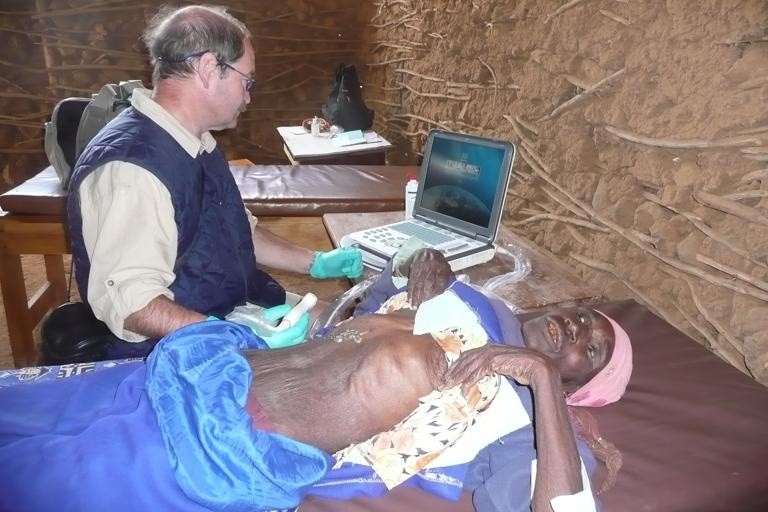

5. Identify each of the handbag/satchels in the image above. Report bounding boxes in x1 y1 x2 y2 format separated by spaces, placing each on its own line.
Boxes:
321 63 374 131
43 78 145 191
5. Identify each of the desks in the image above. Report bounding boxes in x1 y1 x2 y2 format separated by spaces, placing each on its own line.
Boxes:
321 204 603 316
273 119 396 167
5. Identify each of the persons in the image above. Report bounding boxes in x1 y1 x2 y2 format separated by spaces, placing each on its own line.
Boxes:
64 0 364 361
0 246 635 512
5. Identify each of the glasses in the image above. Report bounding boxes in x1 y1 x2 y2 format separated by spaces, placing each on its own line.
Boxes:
176 50 257 92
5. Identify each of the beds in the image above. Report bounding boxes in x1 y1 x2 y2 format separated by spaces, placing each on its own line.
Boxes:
0 152 416 364
0 301 766 511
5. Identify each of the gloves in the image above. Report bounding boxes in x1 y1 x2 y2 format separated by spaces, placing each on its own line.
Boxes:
202 303 311 348
308 244 366 283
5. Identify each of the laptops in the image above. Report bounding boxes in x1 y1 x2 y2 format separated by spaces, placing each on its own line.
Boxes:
340 128 517 273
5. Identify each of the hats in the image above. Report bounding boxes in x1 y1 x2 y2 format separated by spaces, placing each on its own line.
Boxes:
564 308 636 409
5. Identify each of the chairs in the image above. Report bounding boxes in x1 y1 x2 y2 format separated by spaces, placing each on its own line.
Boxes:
36 97 143 363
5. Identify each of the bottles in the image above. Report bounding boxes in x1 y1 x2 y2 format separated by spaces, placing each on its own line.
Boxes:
311 116 320 137
404 177 420 219
